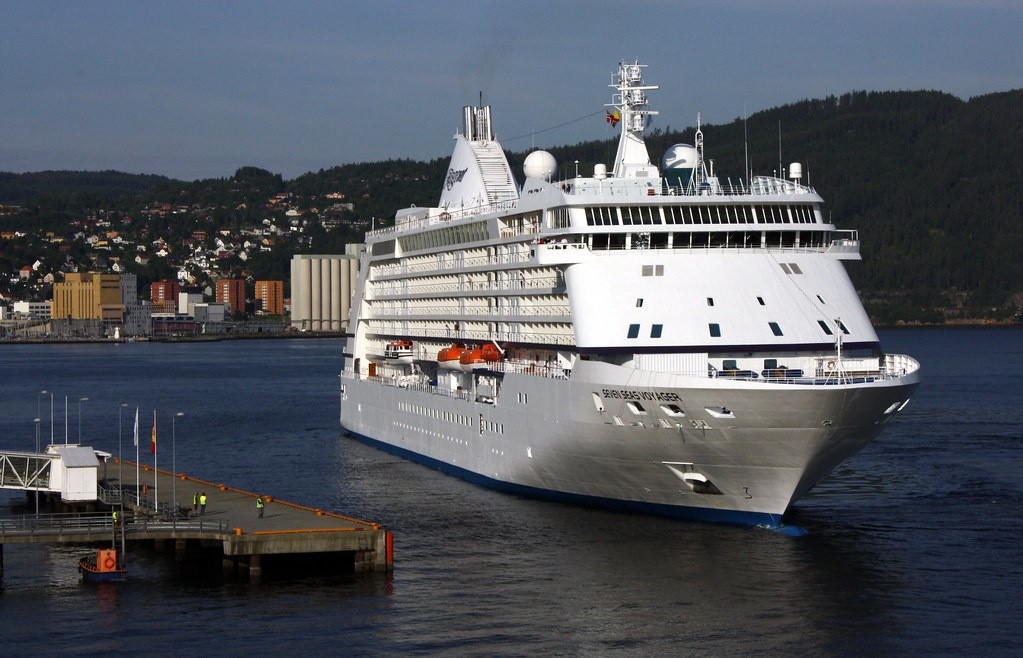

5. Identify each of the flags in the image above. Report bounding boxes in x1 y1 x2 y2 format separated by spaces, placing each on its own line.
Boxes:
607 110 621 127
151 411 155 455
133 405 138 446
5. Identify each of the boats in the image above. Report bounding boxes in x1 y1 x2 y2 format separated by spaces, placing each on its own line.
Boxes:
480 344 504 361
382 340 414 367
437 348 462 371
78 550 128 585
459 349 488 373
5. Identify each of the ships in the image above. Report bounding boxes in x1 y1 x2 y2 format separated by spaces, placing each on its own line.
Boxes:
339 55 923 531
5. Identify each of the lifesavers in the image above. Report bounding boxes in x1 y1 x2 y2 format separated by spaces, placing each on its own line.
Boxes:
440 213 451 221
828 361 836 370
104 557 115 569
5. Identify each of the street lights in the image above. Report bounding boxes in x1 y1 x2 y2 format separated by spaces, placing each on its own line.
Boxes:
118 403 128 511
37 390 47 417
78 397 88 444
173 412 184 516
34 418 42 518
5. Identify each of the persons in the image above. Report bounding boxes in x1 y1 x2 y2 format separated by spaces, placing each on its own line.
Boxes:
257 495 264 519
193 492 199 515
537 236 580 249
199 492 208 516
563 183 570 193
